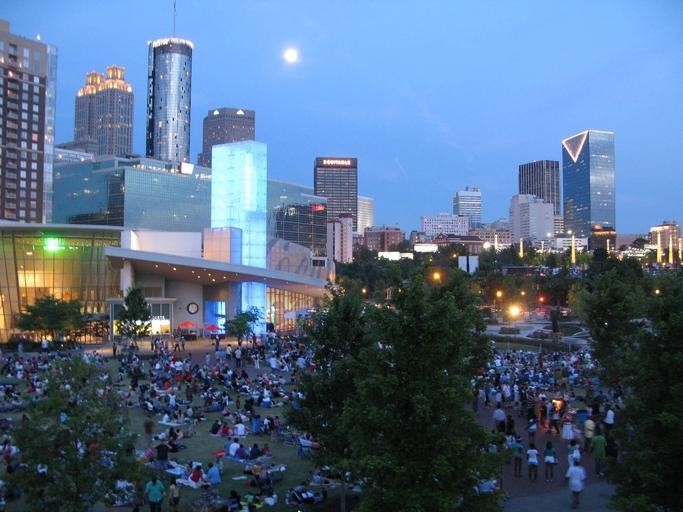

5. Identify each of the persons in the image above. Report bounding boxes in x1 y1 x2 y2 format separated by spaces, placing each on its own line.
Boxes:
449 346 632 508
0 331 370 512
